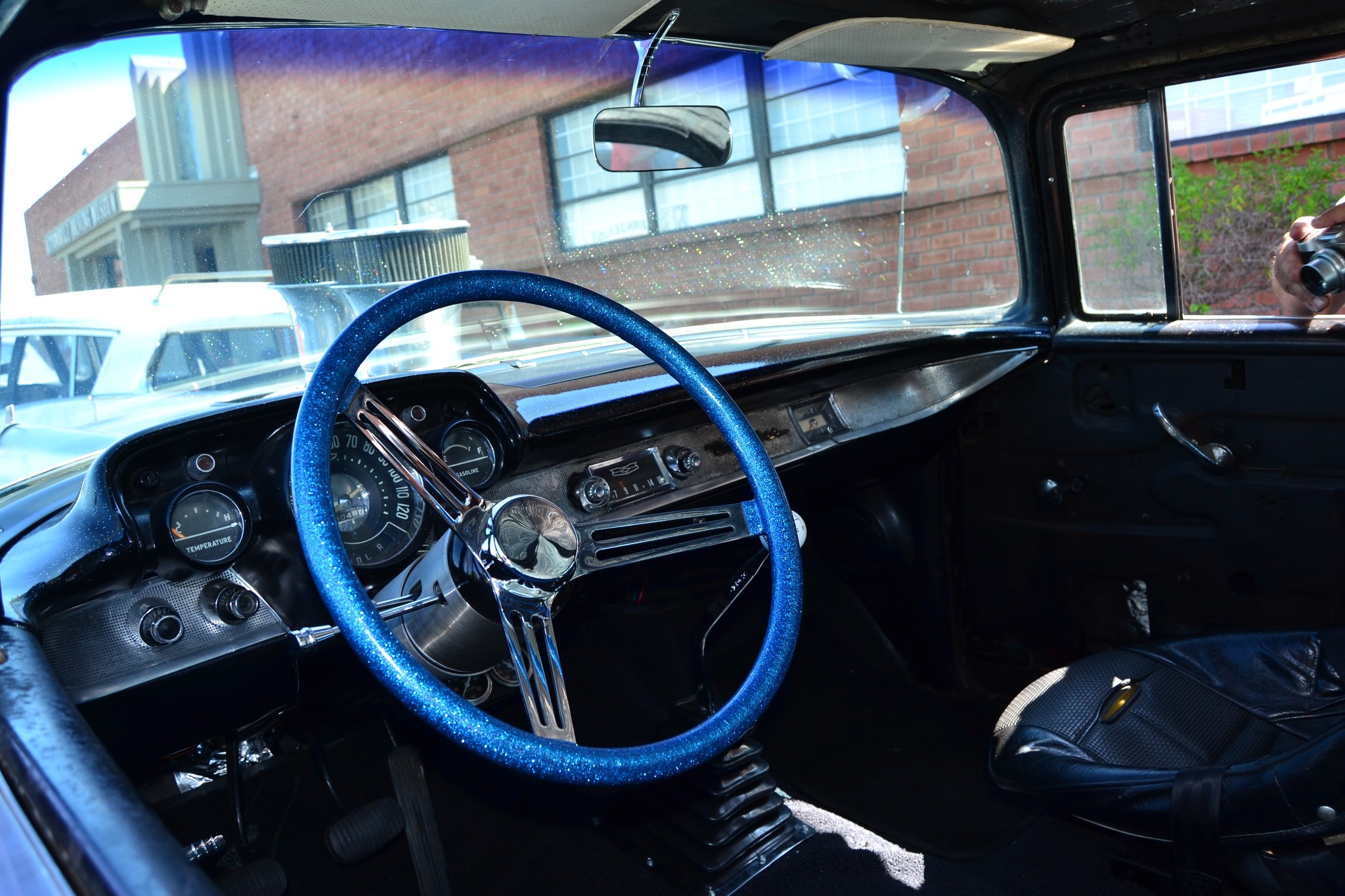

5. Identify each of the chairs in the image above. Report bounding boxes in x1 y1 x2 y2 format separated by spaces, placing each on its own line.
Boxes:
989 626 1345 896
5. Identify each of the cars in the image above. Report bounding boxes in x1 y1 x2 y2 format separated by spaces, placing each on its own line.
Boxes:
2 269 307 484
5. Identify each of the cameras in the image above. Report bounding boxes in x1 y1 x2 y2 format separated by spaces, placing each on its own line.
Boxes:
1296 220 1345 298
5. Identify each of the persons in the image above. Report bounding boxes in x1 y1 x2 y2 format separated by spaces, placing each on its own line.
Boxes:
1269 193 1345 317
